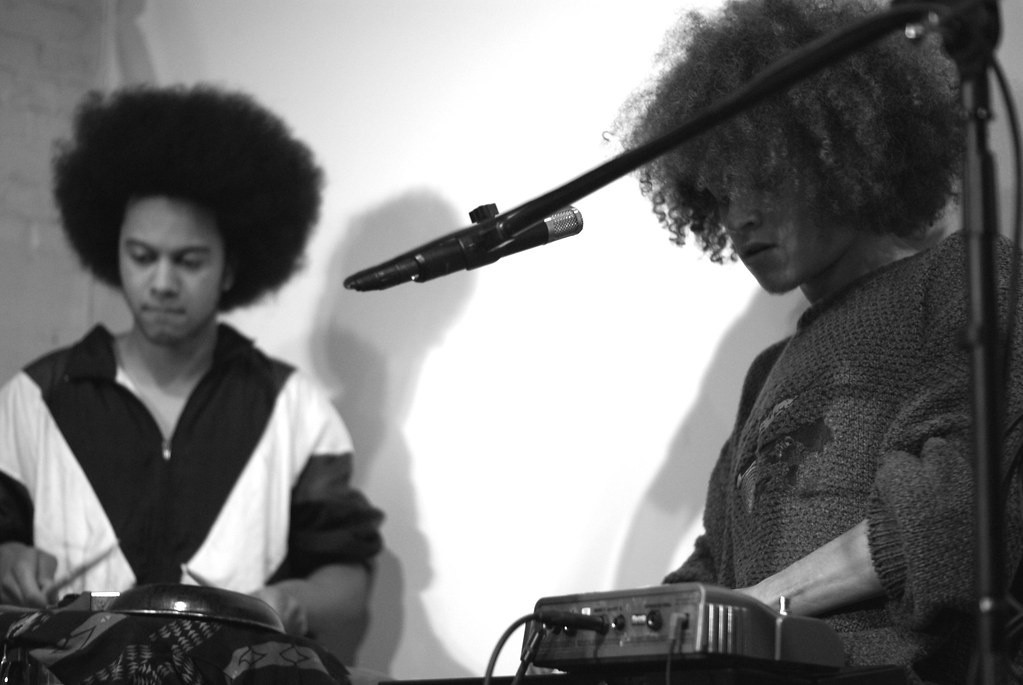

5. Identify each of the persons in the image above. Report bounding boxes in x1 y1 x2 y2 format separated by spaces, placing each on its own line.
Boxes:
0 80 387 639
611 1 1023 685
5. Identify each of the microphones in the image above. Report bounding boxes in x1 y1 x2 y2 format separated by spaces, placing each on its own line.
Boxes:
345 205 584 293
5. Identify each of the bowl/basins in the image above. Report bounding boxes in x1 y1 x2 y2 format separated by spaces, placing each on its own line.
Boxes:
107 584 291 634
